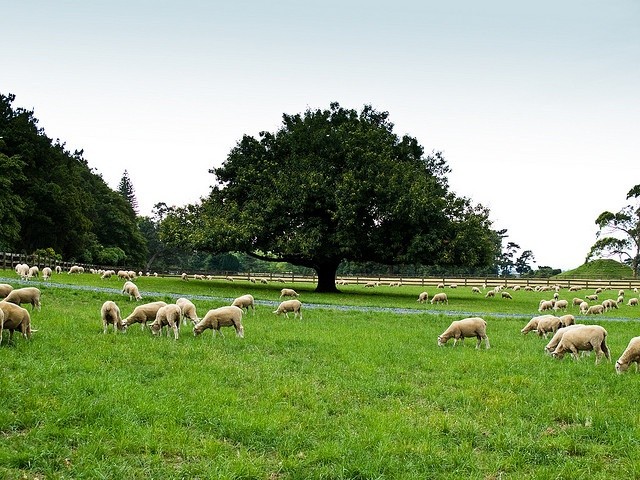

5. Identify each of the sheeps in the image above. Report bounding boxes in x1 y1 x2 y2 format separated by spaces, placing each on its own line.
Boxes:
67 265 78 275
122 280 143 302
148 304 181 340
154 272 158 277
251 277 255 283
602 300 611 313
2 287 42 311
551 325 610 365
146 272 150 276
595 289 602 294
429 293 448 306
626 298 639 307
100 271 111 280
559 315 575 327
55 266 61 274
100 301 128 335
608 298 619 309
193 306 244 338
570 287 577 291
365 282 376 287
552 284 560 291
15 264 22 274
580 301 588 316
577 286 582 290
538 300 553 314
449 285 457 288
0 283 14 300
585 295 598 303
615 336 640 375
0 301 38 344
390 282 402 287
227 276 234 282
585 305 604 316
25 266 39 281
573 298 584 306
138 271 143 277
118 271 136 282
437 284 444 289
521 315 555 336
182 272 188 280
278 279 284 282
261 278 267 284
279 288 299 299
417 292 428 305
472 287 482 294
437 316 490 350
41 267 52 282
90 269 95 274
20 263 30 280
77 267 84 274
524 287 531 291
535 286 551 292
618 296 624 303
194 274 205 279
536 317 563 340
94 269 98 274
554 293 559 300
550 299 556 306
230 294 255 316
485 291 494 298
502 292 513 299
618 290 624 296
123 301 168 332
511 285 520 291
553 300 568 313
272 300 302 320
98 269 101 274
176 298 202 327
102 269 106 274
544 324 591 357
